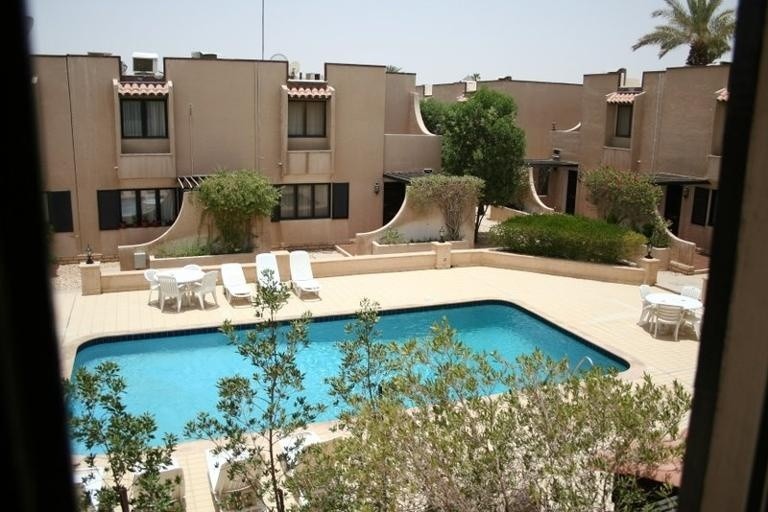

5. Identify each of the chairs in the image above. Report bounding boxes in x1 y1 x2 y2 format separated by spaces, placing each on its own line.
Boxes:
126 457 186 511
288 250 321 299
181 262 202 305
72 461 104 512
219 261 252 305
680 281 702 316
156 274 189 313
254 253 282 296
188 269 220 307
650 301 683 342
280 432 354 505
682 304 702 343
143 267 173 307
205 443 270 510
636 282 667 333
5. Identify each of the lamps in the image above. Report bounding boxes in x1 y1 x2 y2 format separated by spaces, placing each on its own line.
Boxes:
85 243 94 264
374 182 379 193
438 226 445 242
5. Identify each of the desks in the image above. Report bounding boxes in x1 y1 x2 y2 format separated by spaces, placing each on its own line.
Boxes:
648 291 701 336
151 268 207 311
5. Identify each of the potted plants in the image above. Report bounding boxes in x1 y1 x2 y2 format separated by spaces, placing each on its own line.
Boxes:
115 215 173 228
